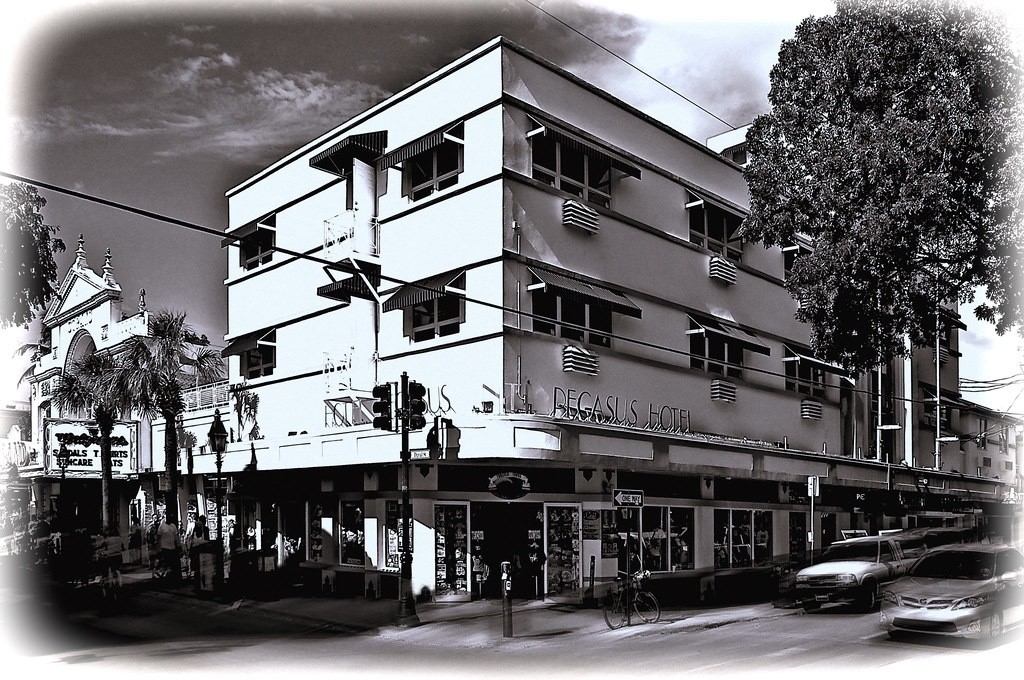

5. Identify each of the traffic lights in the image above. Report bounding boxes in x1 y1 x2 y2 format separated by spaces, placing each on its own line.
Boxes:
372 383 392 431
409 381 426 430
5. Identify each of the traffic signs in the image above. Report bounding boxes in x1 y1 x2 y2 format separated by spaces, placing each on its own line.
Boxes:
612 489 645 509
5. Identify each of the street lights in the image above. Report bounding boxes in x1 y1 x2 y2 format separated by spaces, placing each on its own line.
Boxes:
206 408 230 603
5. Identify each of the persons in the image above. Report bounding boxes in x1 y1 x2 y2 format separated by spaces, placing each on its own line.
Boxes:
3 514 210 590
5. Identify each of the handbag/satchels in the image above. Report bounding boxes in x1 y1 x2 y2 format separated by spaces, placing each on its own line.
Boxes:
177 545 183 558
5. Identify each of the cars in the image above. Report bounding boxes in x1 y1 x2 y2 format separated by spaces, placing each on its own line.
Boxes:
879 543 1024 649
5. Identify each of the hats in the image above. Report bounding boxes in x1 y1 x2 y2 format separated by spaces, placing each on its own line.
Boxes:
199 515 207 520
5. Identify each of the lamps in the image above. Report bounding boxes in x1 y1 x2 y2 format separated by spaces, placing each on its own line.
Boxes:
481 401 493 413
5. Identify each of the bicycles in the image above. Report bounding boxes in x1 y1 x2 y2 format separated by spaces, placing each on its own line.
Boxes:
603 570 660 630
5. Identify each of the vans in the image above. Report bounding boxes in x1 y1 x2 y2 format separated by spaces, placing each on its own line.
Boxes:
922 527 975 549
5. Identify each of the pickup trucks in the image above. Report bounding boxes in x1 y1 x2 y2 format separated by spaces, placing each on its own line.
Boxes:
795 525 928 612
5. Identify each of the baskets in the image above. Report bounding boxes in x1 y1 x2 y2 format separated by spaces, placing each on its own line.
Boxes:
633 578 647 589
